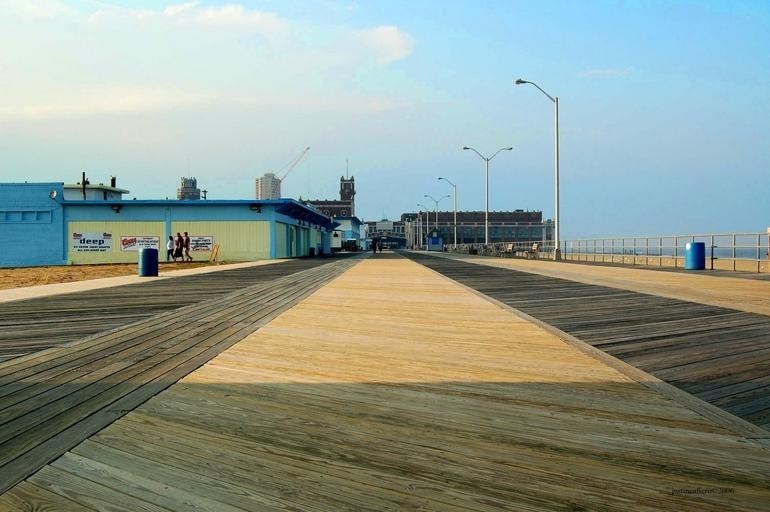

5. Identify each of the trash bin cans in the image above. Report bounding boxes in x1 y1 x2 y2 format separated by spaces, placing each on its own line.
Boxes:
684 242 705 270
310 248 315 258
138 248 158 277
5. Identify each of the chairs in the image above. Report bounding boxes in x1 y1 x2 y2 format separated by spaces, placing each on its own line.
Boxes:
499 242 538 259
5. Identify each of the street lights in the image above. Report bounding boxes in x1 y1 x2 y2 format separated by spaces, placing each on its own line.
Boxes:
461 146 514 246
405 194 450 251
514 79 562 263
438 176 457 249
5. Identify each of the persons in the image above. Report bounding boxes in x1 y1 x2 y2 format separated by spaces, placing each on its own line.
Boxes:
378 241 383 256
444 241 448 251
334 231 338 237
173 233 185 262
183 232 193 262
166 236 176 262
371 238 377 256
